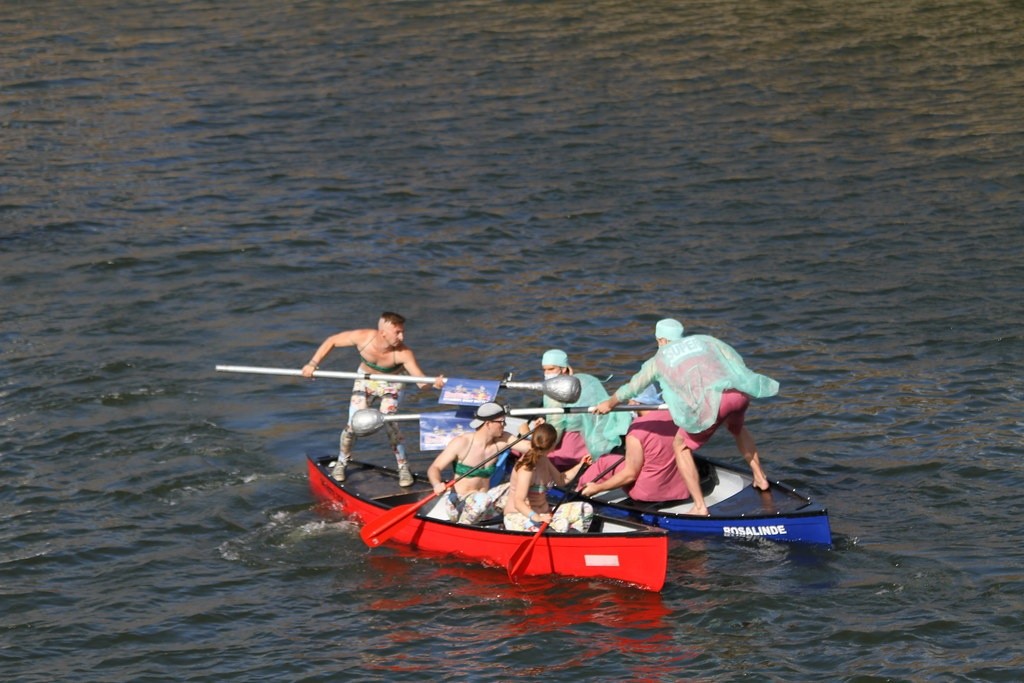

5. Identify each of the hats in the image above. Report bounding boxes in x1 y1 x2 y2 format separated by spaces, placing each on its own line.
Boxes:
542 348 568 368
655 319 684 341
633 384 664 405
469 403 503 429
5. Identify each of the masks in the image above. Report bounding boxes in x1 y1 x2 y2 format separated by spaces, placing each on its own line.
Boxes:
544 371 561 381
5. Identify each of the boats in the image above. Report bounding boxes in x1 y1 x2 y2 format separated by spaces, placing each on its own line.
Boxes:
306 444 670 592
505 453 832 545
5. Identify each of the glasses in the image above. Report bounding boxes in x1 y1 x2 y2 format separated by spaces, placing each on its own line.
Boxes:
491 419 505 426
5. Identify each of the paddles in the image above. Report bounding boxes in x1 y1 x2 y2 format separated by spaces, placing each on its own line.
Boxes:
507 455 593 584
359 424 539 550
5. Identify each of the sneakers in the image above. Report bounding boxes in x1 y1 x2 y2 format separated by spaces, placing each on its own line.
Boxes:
332 460 348 481
397 466 414 487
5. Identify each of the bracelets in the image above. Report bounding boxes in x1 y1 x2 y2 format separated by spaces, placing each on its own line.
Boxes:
307 362 317 370
528 511 536 518
311 359 318 365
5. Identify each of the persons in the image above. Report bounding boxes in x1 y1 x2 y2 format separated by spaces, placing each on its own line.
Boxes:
574 319 780 515
503 423 594 534
427 403 532 528
528 349 631 463
303 311 444 486
